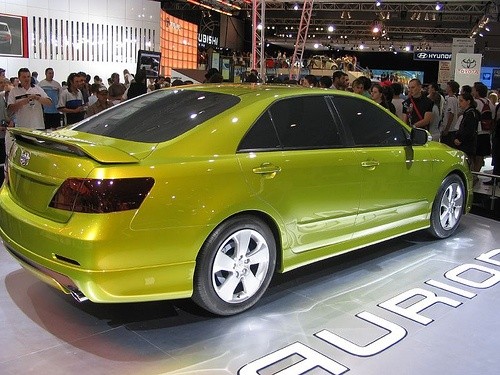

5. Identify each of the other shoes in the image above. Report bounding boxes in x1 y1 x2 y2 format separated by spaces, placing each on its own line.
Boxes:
483 180 498 186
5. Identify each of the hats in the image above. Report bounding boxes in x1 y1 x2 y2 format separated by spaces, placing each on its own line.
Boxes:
97 86 108 94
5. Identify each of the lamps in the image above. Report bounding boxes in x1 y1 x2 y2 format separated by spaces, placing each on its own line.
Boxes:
410 12 435 20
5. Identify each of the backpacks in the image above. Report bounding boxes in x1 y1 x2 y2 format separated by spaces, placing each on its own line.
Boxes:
479 99 493 131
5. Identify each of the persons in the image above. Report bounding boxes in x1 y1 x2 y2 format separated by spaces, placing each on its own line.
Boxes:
0 67 223 189
233 52 500 186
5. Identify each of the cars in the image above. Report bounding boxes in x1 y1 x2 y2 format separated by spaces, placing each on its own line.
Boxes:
0 81 475 319
